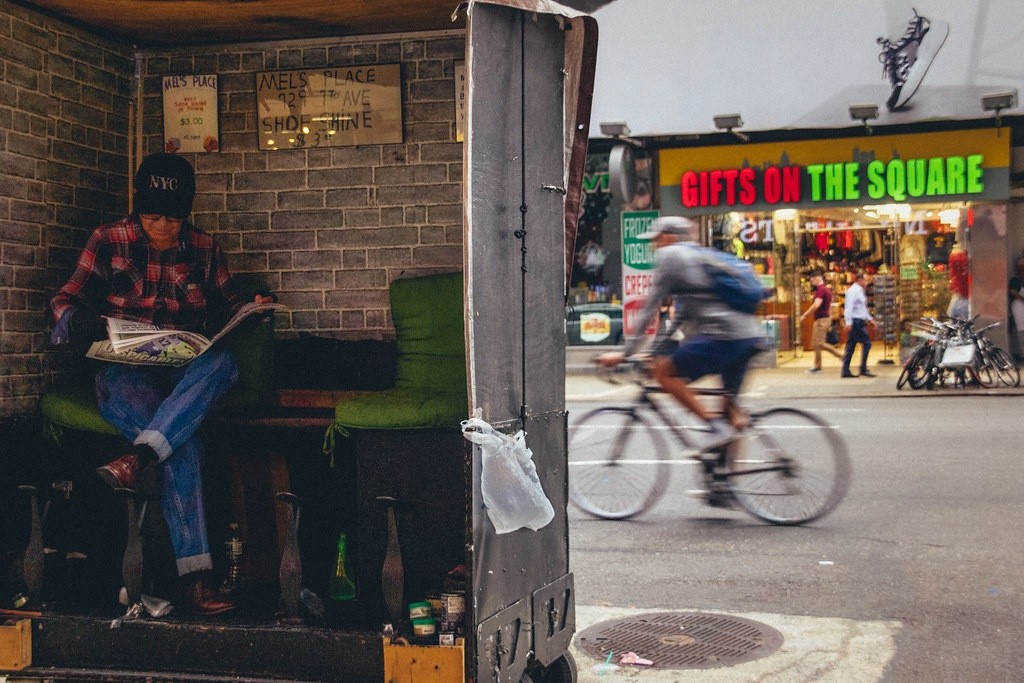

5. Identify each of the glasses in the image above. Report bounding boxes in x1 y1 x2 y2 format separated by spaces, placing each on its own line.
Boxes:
141 213 183 224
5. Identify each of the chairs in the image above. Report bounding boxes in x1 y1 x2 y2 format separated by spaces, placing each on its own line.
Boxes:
330 269 475 619
32 273 308 622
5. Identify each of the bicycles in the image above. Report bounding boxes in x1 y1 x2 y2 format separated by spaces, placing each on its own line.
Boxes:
565 357 850 526
896 312 1021 390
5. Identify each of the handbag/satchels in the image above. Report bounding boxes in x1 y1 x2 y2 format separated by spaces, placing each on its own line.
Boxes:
938 343 976 369
459 408 556 536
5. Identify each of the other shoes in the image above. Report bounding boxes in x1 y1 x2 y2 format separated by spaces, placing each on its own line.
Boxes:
687 488 711 499
700 418 742 453
841 370 858 377
860 369 875 378
809 367 820 371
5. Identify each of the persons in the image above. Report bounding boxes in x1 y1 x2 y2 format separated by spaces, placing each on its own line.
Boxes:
800 270 843 374
49 155 276 614
1008 256 1024 362
840 274 878 379
592 216 767 507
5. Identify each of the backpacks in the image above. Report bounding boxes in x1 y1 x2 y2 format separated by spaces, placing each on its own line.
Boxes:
662 241 764 312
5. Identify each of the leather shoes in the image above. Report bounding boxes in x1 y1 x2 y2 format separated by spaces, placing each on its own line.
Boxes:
98 452 148 495
187 571 240 616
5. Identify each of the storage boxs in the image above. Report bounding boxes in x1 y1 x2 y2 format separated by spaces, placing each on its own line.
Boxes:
380 630 465 683
0 618 33 671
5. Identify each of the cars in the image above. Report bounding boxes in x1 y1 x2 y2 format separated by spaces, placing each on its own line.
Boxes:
566 304 624 346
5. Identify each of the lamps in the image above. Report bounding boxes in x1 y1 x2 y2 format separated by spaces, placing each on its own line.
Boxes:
982 95 1015 127
850 106 882 135
602 123 647 153
713 114 748 143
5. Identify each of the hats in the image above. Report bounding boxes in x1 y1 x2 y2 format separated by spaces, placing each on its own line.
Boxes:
635 216 695 239
135 152 197 218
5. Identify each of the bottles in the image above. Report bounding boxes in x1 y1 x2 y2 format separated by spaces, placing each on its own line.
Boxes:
587 284 619 303
323 532 355 600
224 522 245 584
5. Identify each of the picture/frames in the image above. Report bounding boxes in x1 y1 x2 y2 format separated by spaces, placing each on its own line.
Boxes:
254 61 405 151
